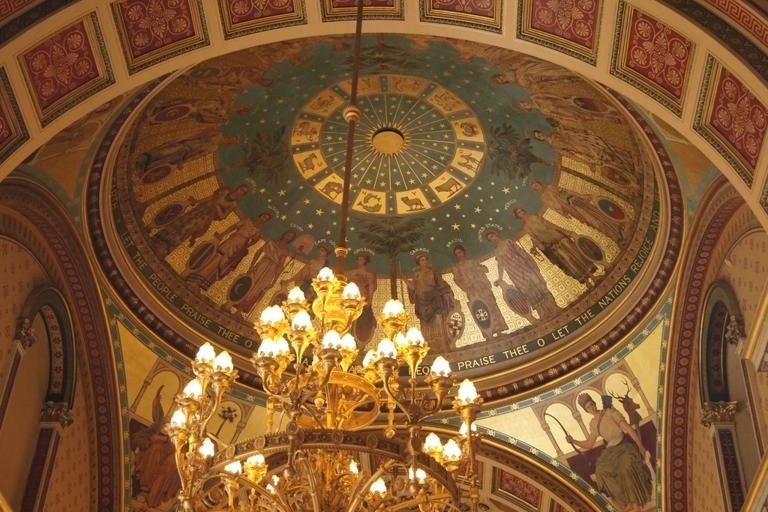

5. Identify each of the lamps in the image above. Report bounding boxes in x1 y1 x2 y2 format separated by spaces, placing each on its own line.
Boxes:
165 1 481 511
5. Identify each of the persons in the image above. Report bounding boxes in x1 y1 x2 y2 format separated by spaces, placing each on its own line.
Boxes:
561 388 656 507
126 381 192 508
